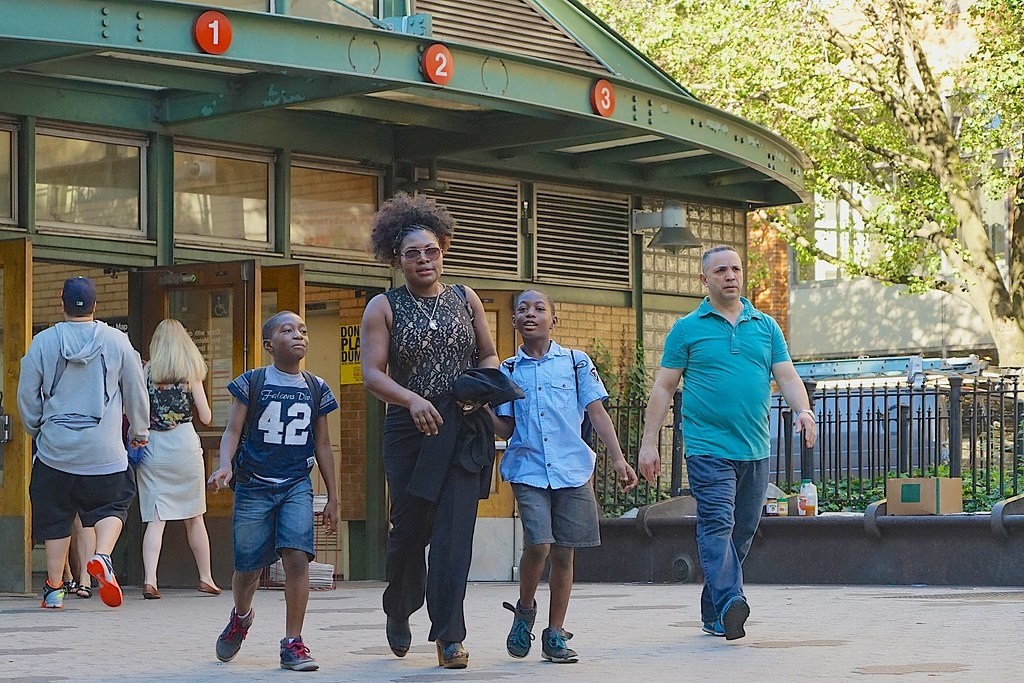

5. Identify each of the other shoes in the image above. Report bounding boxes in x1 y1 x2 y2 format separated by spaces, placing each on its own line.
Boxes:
198 580 221 594
143 584 160 599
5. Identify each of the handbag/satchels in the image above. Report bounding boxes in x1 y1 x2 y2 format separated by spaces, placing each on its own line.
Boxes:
127 440 143 464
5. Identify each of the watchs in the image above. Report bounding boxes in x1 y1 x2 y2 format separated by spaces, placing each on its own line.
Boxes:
796 409 816 421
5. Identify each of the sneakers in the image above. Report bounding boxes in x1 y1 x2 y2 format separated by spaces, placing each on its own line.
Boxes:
720 596 750 640
503 598 537 658
702 621 725 636
541 628 579 662
41 580 65 607
87 554 122 607
280 636 319 670
216 606 255 662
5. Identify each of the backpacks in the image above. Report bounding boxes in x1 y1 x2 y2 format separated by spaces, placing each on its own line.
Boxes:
571 348 594 448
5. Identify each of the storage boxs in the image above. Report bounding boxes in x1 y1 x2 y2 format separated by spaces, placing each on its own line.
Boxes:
886 475 964 516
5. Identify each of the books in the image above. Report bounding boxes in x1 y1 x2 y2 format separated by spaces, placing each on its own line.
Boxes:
269 558 335 588
312 495 327 513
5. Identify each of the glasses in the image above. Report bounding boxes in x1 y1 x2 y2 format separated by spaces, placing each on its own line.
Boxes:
398 247 444 263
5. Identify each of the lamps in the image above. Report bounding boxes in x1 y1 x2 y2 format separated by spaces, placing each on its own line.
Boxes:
632 198 702 257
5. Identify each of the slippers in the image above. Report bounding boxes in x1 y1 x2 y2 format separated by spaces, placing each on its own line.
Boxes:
76 585 92 598
66 579 78 594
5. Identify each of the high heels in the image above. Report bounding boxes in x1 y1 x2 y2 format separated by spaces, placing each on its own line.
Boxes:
386 616 411 657
436 639 469 668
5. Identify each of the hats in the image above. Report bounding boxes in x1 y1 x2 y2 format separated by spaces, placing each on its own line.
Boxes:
62 277 95 307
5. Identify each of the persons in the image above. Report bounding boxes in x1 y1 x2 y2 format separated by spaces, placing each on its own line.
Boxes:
638 245 817 641
16 277 150 609
482 289 636 664
127 317 222 599
208 311 339 671
358 192 500 668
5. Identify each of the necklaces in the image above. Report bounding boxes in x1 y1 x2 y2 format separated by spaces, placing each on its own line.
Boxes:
405 284 442 330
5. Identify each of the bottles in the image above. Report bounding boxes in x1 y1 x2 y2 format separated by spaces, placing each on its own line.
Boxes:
798 479 818 516
777 499 788 516
766 498 777 516
